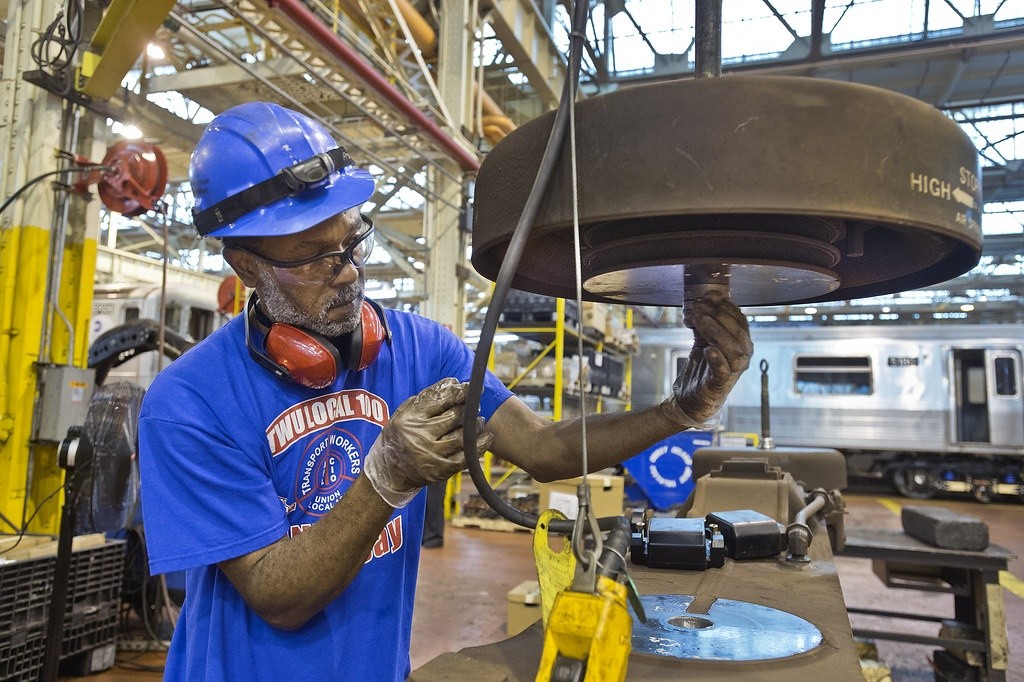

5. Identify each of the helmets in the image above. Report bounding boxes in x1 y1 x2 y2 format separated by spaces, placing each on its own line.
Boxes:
190 102 374 237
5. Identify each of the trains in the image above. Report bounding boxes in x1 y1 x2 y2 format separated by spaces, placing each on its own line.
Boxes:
631 321 1024 507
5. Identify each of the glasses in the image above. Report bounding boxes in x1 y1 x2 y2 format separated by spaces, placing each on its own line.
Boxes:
223 214 377 285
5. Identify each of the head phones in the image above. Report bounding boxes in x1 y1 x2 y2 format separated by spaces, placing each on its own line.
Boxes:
243 287 393 389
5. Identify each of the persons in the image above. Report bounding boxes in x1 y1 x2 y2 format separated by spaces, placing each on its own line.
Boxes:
137 100 755 680
